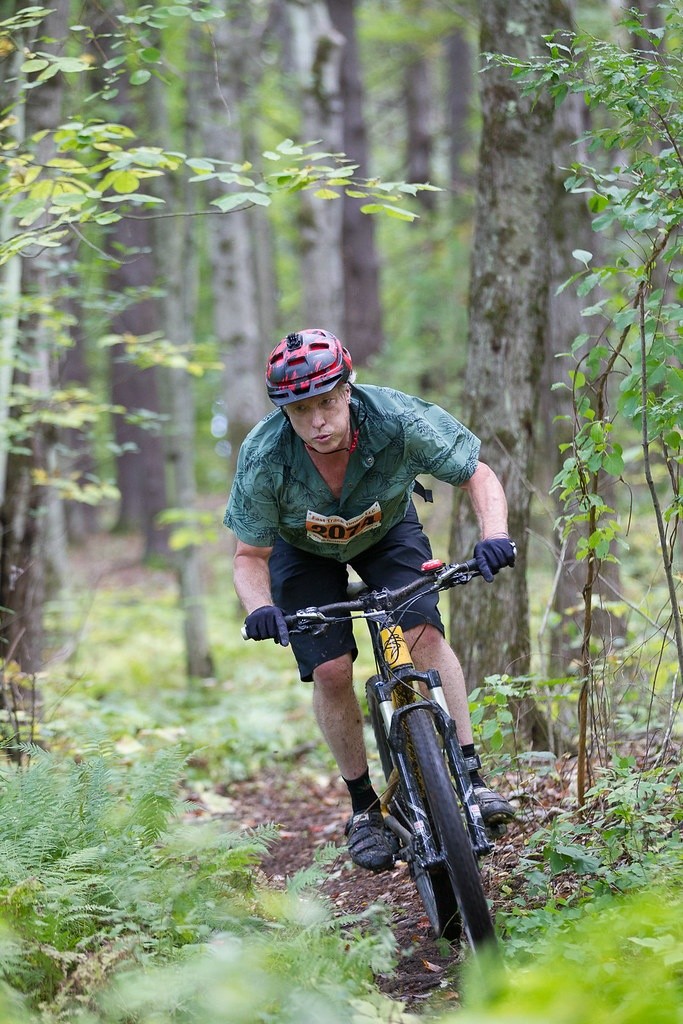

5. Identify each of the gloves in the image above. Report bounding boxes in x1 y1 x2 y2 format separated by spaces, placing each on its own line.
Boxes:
245 604 290 647
473 532 517 583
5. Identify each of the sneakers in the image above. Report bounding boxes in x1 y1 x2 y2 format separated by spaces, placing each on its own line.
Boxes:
470 782 515 826
345 810 393 870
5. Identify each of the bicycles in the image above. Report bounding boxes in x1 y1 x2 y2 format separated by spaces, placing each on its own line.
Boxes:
240 539 521 950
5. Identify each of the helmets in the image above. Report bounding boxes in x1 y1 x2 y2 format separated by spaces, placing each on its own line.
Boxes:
265 329 351 407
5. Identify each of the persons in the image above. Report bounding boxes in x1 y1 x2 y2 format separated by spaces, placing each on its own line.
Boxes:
221 329 516 871
